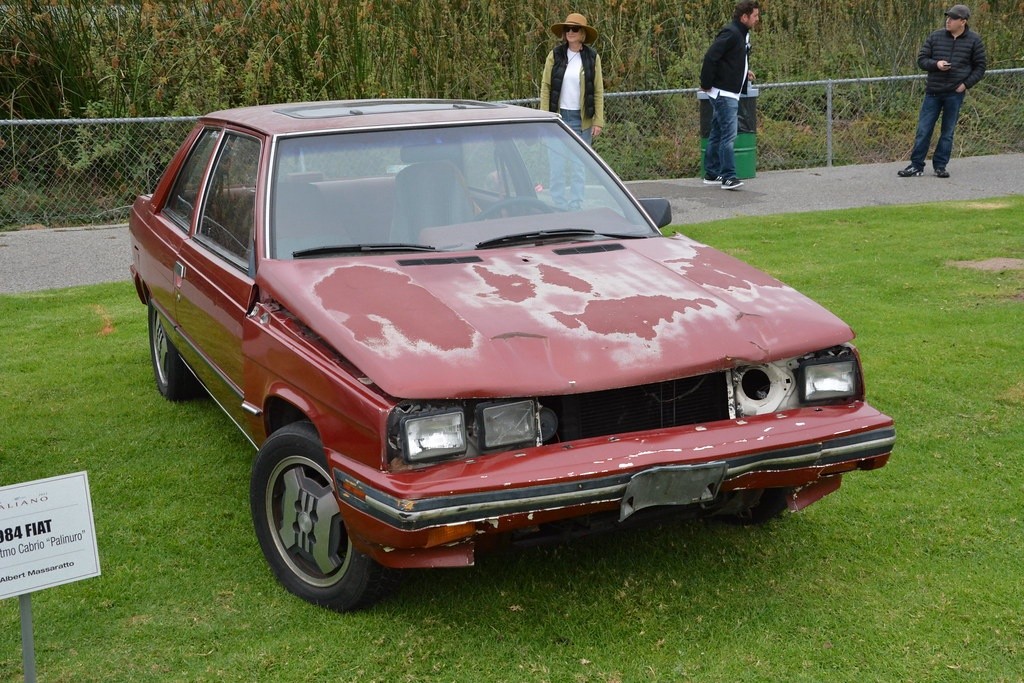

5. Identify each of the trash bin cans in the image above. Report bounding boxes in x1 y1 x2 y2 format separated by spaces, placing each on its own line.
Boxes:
693 87 758 179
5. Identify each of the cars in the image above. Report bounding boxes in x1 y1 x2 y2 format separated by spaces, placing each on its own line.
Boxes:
127 99 894 621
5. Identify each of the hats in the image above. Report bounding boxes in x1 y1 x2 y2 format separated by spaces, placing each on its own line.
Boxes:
944 4 970 19
551 12 597 44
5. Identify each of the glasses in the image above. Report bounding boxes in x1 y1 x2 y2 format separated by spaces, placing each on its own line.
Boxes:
560 26 582 32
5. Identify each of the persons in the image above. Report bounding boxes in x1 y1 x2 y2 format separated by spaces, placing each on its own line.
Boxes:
537 13 606 212
896 5 987 178
700 1 763 189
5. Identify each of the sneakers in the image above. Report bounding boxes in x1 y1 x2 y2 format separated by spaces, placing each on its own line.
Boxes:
703 172 725 184
897 163 925 176
721 177 744 189
931 164 950 177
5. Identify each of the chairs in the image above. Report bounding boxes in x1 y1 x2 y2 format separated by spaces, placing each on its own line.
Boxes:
180 157 493 272
